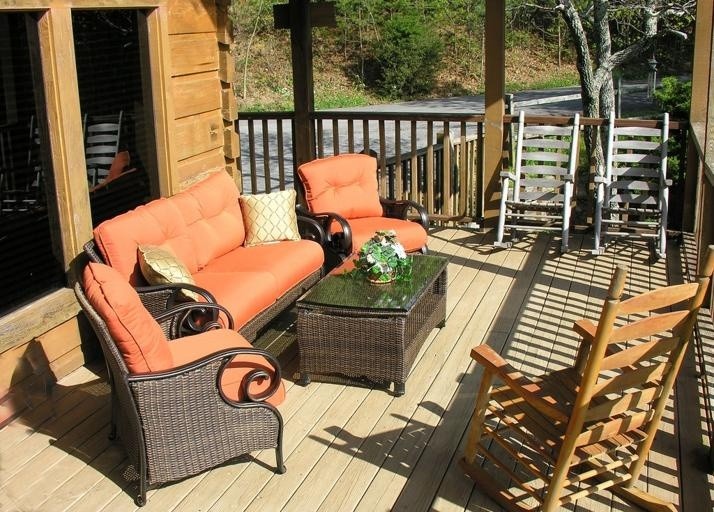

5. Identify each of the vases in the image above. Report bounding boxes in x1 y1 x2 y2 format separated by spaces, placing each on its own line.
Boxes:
364 271 395 284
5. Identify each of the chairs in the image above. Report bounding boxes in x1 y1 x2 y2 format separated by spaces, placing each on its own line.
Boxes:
491 110 673 260
458 245 714 512
70 261 286 508
295 153 428 265
0 108 132 216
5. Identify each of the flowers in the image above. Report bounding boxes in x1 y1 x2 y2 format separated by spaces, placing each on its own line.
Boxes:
353 230 412 283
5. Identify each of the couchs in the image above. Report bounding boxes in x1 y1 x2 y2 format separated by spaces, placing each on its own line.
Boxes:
82 168 328 343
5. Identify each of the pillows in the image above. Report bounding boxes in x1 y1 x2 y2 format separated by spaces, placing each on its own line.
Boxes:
135 244 200 303
238 188 302 248
